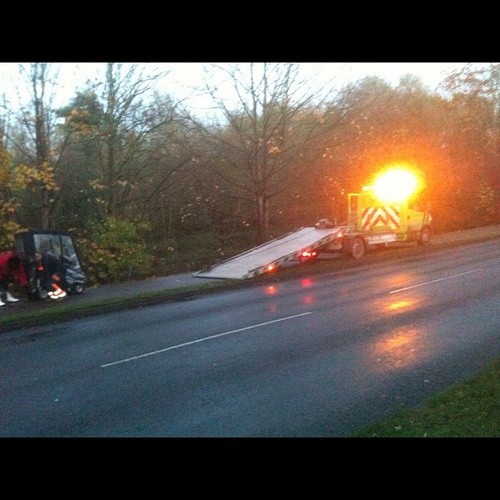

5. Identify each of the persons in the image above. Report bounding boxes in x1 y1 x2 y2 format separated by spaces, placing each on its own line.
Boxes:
0 252 29 309
32 251 74 294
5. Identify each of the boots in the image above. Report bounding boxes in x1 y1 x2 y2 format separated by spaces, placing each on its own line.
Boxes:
0 298 6 306
6 291 20 302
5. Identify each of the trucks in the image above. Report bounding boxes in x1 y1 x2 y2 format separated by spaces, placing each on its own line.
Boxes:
191 189 433 280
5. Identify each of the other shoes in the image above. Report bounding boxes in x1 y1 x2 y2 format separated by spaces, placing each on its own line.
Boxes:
49 288 67 299
